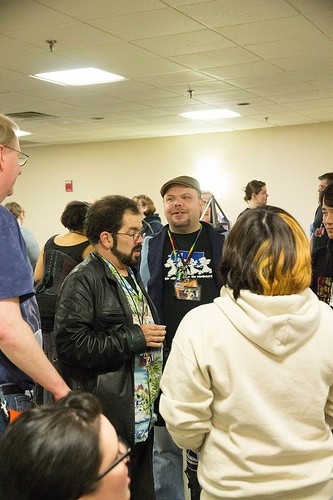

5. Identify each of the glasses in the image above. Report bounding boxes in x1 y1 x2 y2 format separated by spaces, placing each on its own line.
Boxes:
99 446 132 488
115 228 145 240
2 145 29 166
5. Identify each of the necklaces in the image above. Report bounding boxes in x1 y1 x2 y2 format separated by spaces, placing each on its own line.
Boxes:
96 251 152 367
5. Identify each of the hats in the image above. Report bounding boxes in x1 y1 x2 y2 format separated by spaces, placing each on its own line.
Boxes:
160 175 200 197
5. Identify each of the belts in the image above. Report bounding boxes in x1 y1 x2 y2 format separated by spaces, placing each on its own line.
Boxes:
2 384 26 394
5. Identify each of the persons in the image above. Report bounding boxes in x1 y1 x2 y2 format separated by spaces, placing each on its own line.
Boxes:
55 196 167 500
0 389 134 500
159 206 333 500
0 112 76 432
136 176 226 500
4 170 333 306
